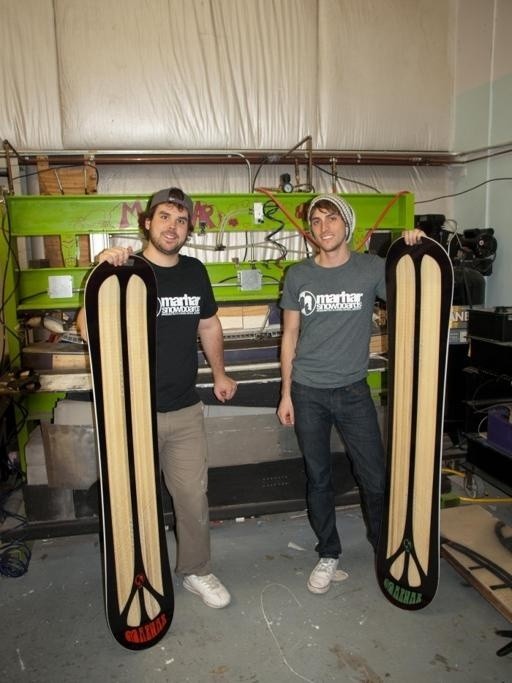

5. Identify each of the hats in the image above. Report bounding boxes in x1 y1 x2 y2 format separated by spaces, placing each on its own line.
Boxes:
149 188 193 218
308 194 356 244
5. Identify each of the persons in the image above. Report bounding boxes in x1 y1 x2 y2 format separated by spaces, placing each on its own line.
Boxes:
76 188 238 609
277 194 427 594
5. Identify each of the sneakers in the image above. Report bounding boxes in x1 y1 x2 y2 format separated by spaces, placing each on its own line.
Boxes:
183 574 231 610
307 555 339 595
